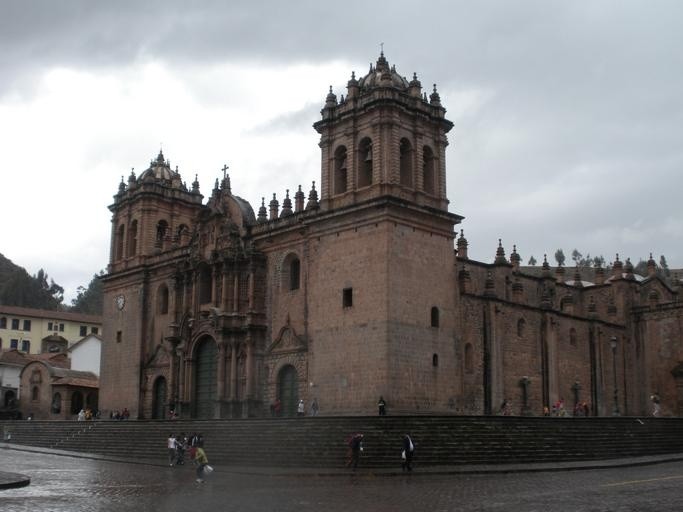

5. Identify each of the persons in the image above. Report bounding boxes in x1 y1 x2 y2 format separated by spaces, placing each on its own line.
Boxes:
166 430 208 486
399 434 413 472
540 394 590 418
76 405 130 421
344 435 361 473
296 399 304 417
272 398 281 417
499 398 507 416
377 395 387 416
310 396 318 417
649 392 660 417
503 397 513 416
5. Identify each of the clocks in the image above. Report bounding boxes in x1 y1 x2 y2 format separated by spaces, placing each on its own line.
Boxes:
116 293 125 311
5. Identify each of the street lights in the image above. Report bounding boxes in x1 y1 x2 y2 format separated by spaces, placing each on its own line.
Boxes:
609 336 619 417
